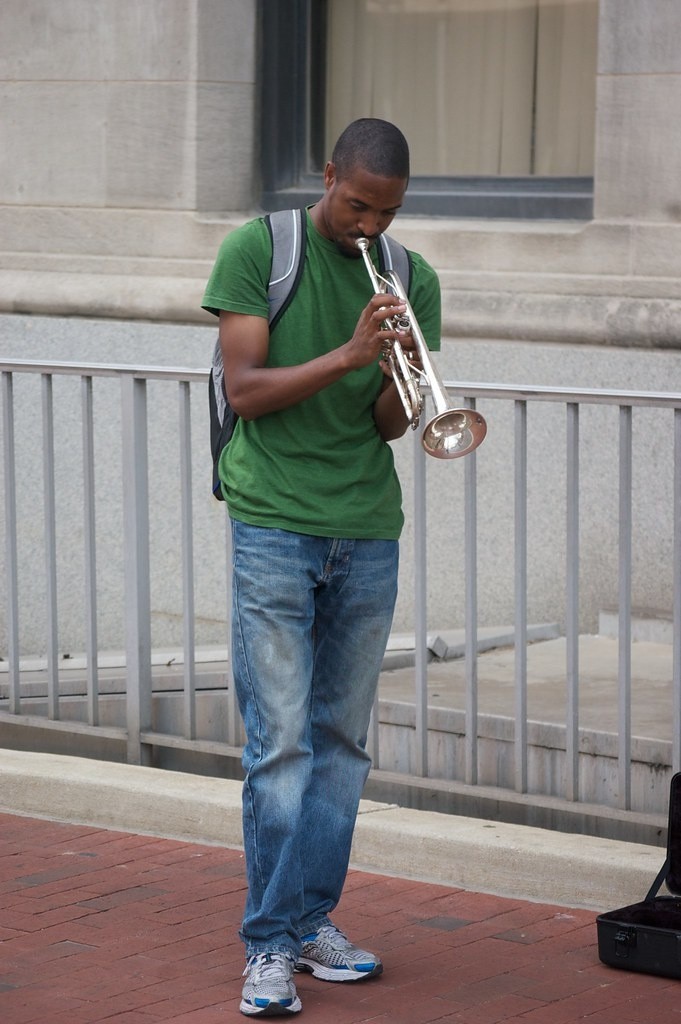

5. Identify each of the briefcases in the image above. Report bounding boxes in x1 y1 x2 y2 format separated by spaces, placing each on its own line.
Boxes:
596 771 680 981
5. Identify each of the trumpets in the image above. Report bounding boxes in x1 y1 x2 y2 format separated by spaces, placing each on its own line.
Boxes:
354 236 488 460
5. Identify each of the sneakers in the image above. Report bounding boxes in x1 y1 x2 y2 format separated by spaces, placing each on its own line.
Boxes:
293 924 382 982
237 954 302 1017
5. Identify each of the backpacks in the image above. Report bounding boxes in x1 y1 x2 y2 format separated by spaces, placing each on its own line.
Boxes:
208 209 414 501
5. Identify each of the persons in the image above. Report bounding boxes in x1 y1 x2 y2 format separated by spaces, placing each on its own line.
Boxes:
196 116 442 1019
